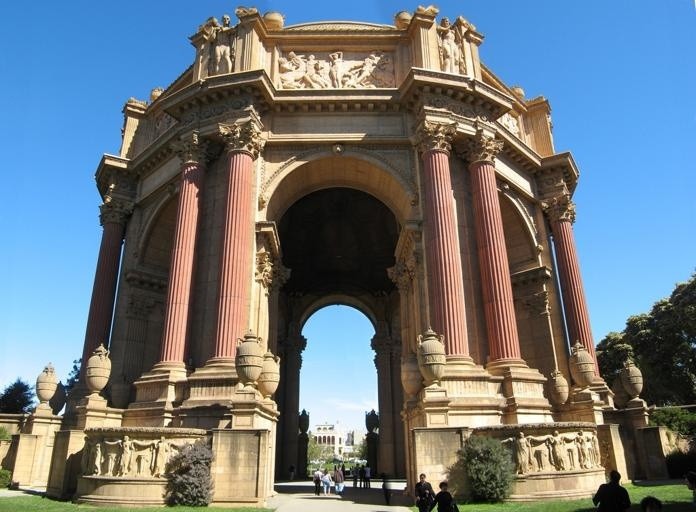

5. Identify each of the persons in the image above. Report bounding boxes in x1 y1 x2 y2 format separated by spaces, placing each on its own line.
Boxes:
574 430 589 471
115 435 136 479
311 466 322 496
351 464 360 488
75 435 97 475
321 468 332 496
591 470 631 511
682 471 695 511
276 47 394 89
206 9 242 76
638 495 662 512
381 479 391 506
413 473 436 512
427 482 455 512
150 435 170 479
505 431 531 477
591 430 605 467
363 463 371 489
333 465 344 495
438 18 465 74
547 430 571 471
358 465 367 488
